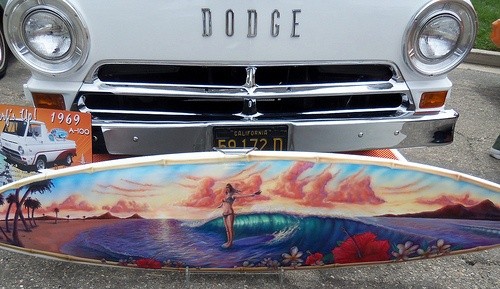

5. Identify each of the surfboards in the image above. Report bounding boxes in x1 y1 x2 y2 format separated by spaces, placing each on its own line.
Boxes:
0 146 500 278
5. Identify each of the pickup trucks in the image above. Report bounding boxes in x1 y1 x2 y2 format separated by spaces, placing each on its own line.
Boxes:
2 118 77 171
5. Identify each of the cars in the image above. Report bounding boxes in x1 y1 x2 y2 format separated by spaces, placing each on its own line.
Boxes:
4 2 478 155
